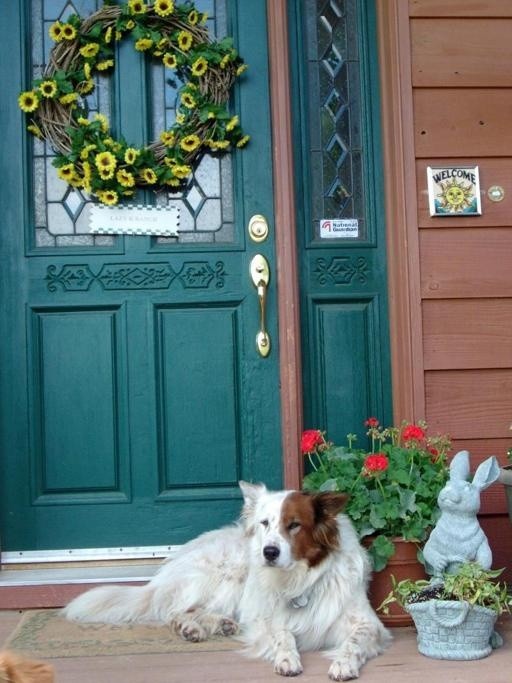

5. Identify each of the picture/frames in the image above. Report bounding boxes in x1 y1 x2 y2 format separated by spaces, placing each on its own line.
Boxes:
427 164 482 217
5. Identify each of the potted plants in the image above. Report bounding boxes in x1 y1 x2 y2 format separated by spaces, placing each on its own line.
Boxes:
377 562 510 659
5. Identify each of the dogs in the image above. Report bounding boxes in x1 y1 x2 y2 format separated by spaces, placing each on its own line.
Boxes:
61 478 392 682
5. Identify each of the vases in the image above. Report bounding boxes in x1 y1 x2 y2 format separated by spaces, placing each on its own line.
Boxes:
357 536 429 626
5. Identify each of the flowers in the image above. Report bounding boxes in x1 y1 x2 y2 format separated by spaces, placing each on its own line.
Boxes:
17 1 251 207
302 421 450 536
